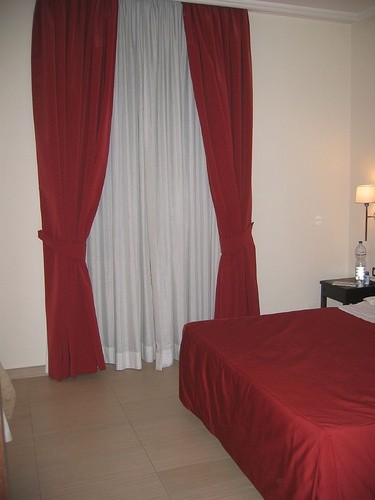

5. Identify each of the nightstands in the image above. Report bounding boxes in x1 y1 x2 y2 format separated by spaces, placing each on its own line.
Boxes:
320 277 375 309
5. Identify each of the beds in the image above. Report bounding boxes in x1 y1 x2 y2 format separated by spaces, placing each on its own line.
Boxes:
178 296 375 500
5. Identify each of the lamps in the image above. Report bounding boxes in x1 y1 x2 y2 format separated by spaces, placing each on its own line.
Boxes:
355 184 375 241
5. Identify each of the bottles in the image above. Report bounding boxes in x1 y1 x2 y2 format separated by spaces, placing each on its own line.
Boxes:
365 272 369 284
354 241 367 281
